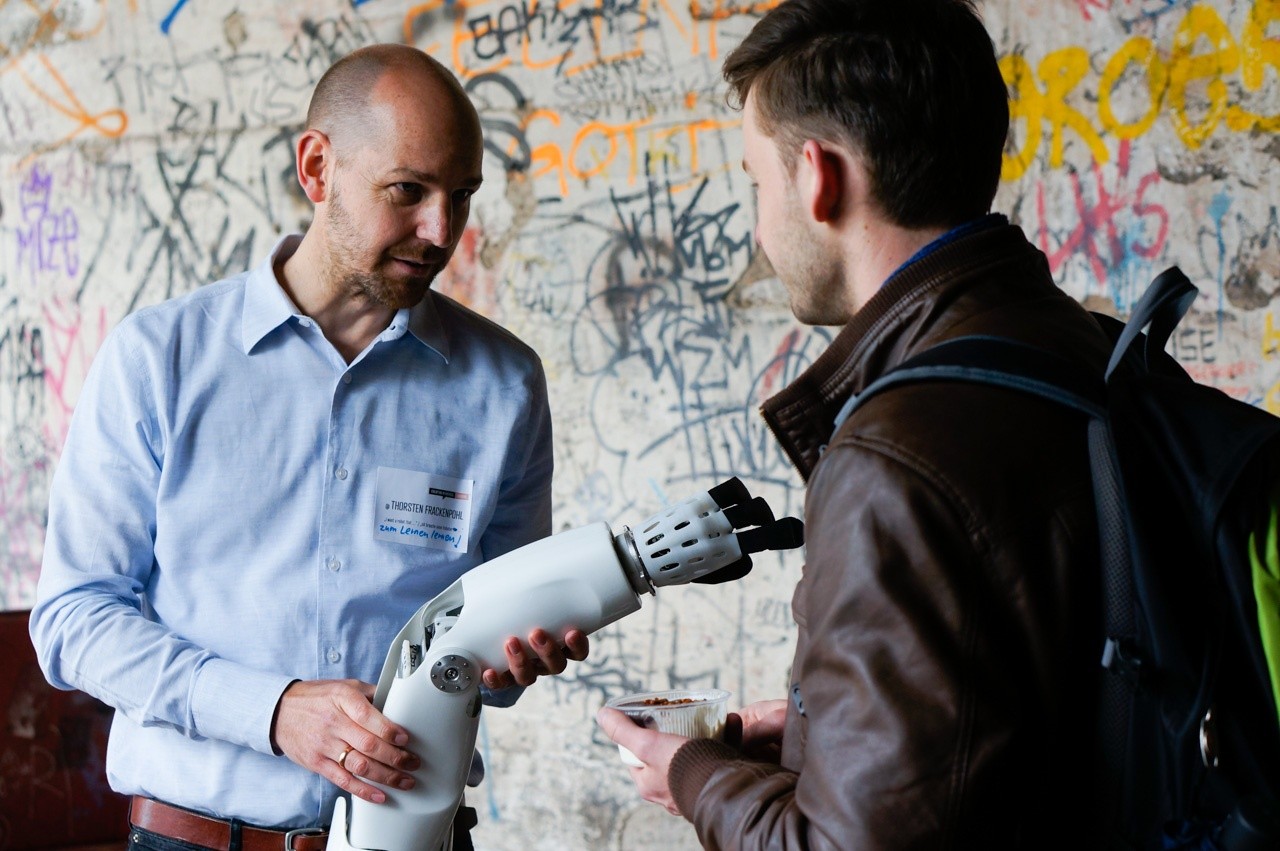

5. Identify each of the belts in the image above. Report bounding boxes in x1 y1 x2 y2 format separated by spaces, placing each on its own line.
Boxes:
129 794 477 851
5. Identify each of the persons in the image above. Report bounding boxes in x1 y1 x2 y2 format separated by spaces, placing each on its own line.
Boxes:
590 3 1280 850
25 42 557 851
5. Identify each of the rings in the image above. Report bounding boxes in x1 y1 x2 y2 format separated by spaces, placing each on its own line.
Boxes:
337 745 355 771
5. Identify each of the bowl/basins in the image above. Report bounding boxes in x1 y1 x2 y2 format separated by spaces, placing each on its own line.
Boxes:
607 685 734 770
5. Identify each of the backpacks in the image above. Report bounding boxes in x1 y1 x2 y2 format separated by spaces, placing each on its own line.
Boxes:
817 265 1280 851
